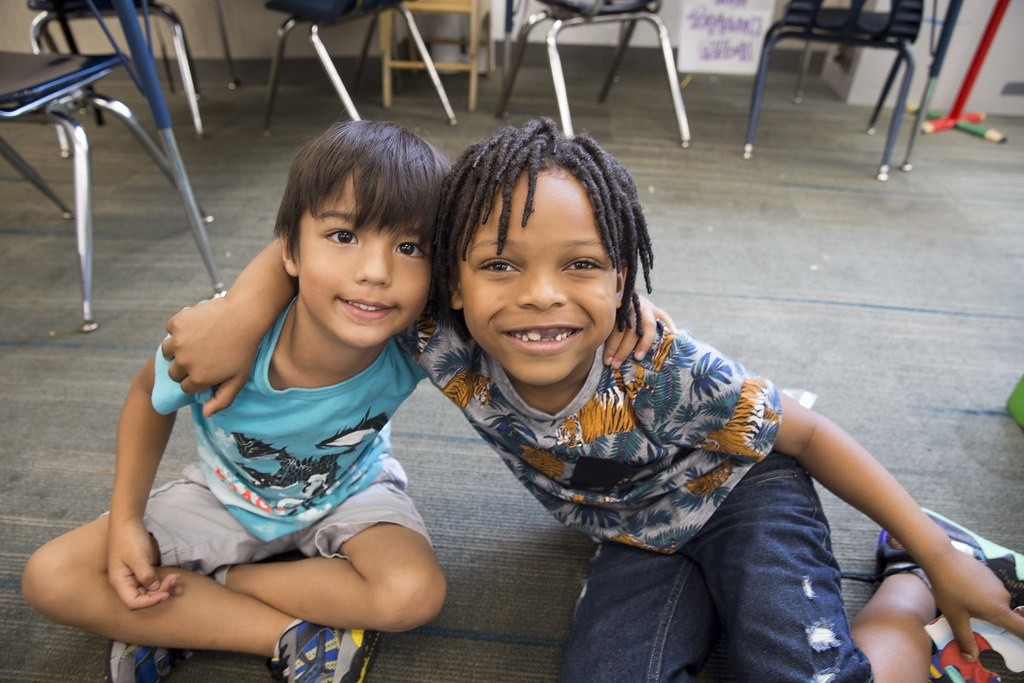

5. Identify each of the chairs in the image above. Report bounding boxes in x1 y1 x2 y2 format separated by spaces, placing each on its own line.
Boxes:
27 0 208 159
743 0 924 182
0 52 215 331
263 0 457 136
379 0 494 112
496 0 691 148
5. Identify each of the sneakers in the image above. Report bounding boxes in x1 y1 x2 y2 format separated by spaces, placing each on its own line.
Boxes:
875 505 1024 610
103 532 217 683
923 613 1024 683
272 619 380 683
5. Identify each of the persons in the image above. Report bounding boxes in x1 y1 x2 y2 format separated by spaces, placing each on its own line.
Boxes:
19 121 675 682
160 116 1024 682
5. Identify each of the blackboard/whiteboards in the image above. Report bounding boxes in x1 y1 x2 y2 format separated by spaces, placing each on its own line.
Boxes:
677 0 778 76
